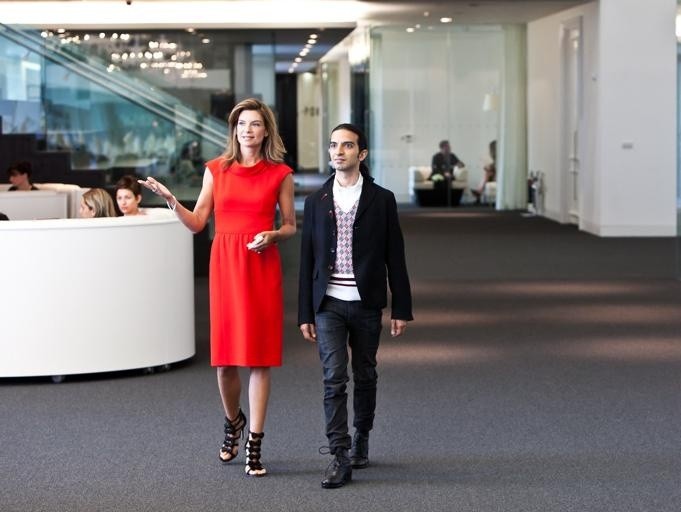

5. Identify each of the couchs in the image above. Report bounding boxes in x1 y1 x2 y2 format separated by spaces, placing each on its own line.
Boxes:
0 178 199 388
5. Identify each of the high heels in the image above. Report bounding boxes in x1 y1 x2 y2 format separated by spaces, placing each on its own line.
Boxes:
219 408 246 462
244 430 266 477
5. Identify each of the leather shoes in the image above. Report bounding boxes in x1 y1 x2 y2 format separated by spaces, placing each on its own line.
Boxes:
321 459 352 488
351 434 368 468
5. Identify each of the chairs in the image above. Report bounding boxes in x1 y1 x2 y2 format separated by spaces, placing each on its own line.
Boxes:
411 165 469 207
478 179 497 207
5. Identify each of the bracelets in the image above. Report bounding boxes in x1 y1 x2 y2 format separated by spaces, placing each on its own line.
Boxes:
165 194 176 212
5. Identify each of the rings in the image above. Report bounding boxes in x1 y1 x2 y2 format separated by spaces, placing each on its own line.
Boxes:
151 187 156 193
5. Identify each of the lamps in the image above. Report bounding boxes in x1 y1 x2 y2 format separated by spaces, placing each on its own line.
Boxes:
481 73 501 113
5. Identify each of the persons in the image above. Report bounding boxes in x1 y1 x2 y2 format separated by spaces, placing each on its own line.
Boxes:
135 97 298 478
187 141 208 177
77 187 117 218
296 123 415 488
470 140 495 207
6 160 38 190
113 174 146 216
427 140 465 207
